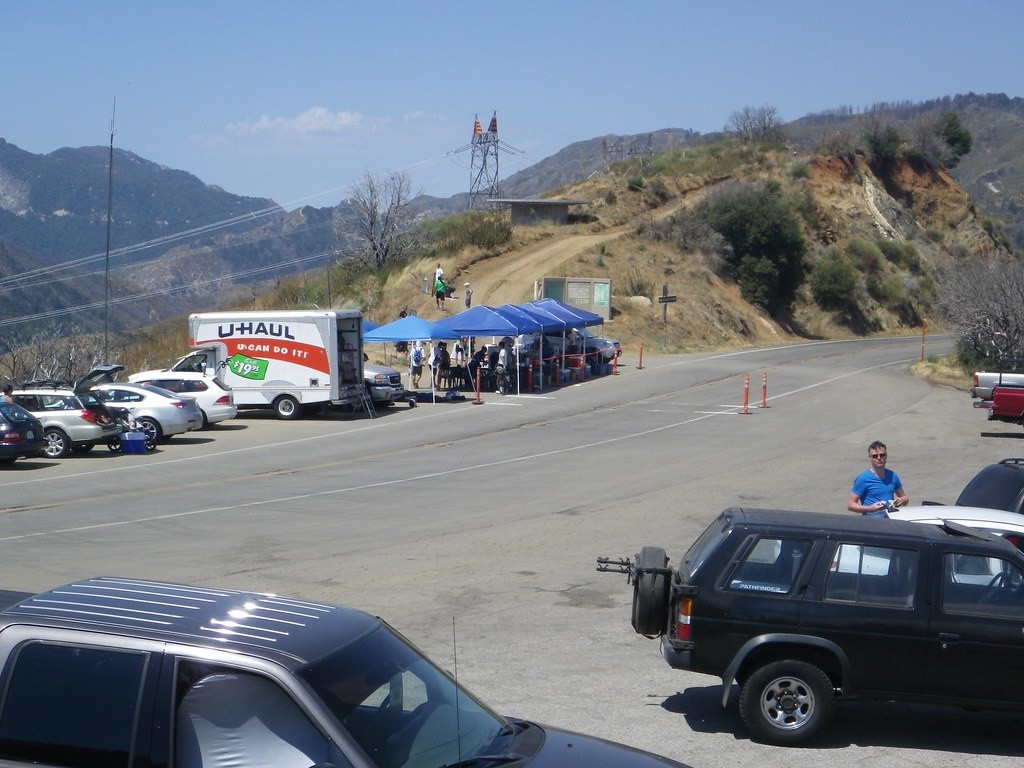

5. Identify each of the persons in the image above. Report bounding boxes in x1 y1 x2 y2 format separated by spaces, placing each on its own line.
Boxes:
435 263 443 282
453 337 467 366
469 346 487 392
427 341 444 389
499 335 515 362
435 277 445 311
3 385 13 402
434 341 451 390
495 341 508 394
847 441 909 519
464 281 473 309
399 305 408 318
463 337 476 358
410 341 426 389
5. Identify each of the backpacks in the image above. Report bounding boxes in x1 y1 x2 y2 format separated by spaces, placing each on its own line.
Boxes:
414 347 423 362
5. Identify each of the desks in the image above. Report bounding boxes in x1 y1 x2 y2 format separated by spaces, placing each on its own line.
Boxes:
433 366 490 389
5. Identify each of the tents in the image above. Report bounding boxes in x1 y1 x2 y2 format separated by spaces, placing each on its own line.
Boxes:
362 297 606 408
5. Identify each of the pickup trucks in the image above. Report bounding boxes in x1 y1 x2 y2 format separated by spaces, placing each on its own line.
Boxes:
972 384 1024 429
971 366 1024 401
1 576 697 768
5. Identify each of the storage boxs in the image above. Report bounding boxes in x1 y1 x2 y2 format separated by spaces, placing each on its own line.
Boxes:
559 356 591 384
119 432 145 454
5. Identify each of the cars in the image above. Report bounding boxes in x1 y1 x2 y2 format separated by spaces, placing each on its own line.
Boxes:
108 353 237 432
0 403 45 466
46 382 204 442
834 507 1024 590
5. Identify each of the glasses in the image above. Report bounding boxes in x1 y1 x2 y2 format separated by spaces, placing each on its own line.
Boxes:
873 454 885 457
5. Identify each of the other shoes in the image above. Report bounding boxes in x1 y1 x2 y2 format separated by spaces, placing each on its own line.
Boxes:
431 385 440 390
496 391 503 394
413 383 419 390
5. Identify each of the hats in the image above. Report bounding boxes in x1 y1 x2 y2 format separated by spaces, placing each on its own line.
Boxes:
463 282 470 285
402 306 407 308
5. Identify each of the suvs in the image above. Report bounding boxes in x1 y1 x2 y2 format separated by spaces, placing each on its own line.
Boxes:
959 458 1024 516
519 328 623 364
627 507 1024 746
349 363 404 413
0 363 125 459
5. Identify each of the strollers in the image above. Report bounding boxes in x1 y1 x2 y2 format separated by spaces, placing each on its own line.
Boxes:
109 408 157 452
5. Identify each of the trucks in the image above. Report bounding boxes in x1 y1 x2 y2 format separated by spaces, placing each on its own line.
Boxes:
128 308 365 420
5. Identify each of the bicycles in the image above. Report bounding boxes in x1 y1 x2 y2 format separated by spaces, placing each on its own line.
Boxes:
495 366 518 395
